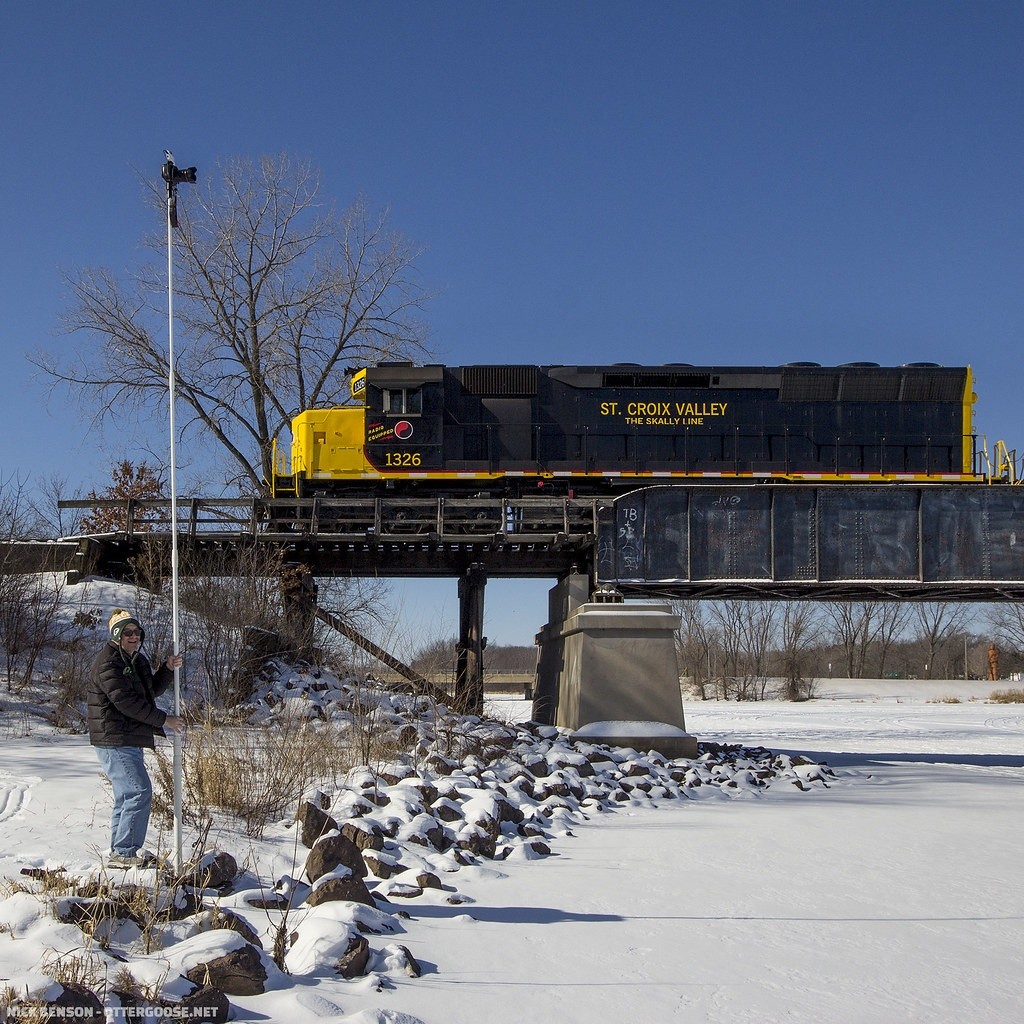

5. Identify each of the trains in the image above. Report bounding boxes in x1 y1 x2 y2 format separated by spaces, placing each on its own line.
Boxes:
260 360 1024 533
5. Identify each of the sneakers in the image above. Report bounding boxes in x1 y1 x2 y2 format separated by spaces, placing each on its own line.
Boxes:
108 852 146 869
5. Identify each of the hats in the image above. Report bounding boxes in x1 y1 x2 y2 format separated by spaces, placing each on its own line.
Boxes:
108 608 146 677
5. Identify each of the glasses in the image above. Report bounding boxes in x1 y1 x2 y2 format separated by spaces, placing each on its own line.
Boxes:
121 628 143 637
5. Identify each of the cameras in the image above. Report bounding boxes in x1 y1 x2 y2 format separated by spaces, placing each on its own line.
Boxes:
161 164 197 186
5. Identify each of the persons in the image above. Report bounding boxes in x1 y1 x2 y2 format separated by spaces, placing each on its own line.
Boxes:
87 609 186 872
